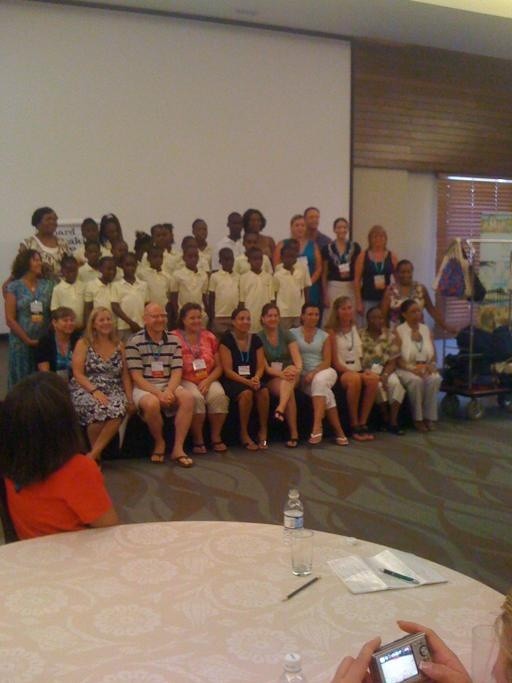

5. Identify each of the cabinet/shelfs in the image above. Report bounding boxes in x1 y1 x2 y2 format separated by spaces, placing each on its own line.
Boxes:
438 240 511 398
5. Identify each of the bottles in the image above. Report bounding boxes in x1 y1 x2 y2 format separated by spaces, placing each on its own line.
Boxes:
279 653 308 683
283 489 303 548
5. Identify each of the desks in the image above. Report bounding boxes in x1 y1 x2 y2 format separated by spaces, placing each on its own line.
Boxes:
1 522 512 683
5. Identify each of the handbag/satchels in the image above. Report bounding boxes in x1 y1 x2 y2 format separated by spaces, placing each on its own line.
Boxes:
444 293 478 327
436 257 485 303
432 327 512 386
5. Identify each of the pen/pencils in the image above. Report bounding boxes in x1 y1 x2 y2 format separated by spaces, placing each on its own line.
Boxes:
378 568 420 584
283 575 321 601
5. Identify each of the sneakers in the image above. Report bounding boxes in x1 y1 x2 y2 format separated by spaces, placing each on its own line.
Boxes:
416 419 435 432
379 420 406 435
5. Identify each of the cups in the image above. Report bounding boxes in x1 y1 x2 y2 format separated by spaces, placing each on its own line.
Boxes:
290 529 313 577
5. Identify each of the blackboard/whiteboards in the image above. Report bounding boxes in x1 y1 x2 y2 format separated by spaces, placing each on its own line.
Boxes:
54 219 102 253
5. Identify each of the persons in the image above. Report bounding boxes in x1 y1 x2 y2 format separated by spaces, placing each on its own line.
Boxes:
332 586 512 683
4 207 462 467
3 371 118 544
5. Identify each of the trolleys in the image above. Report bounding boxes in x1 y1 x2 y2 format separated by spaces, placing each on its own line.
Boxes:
433 238 512 421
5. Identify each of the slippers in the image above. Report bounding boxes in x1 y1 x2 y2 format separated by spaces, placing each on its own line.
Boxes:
210 440 228 453
288 438 298 447
174 454 194 468
274 409 286 423
194 443 207 455
244 439 269 450
336 434 349 445
309 433 323 444
350 425 375 441
151 452 165 463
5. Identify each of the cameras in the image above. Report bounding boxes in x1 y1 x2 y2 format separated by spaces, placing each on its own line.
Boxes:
369 630 435 683
